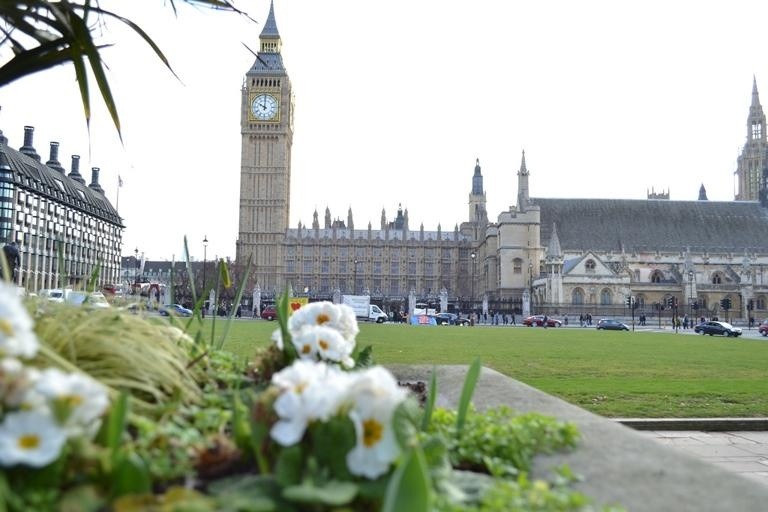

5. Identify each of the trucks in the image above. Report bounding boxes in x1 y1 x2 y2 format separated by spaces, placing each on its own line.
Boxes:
341 295 388 323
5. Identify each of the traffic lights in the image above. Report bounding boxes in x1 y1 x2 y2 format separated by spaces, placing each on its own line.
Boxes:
626 297 632 307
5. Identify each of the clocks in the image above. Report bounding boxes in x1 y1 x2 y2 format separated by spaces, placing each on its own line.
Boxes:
247 87 281 124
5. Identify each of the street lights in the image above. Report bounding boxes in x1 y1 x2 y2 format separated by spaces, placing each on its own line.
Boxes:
354 259 358 296
470 252 475 297
529 263 532 314
689 270 693 330
201 236 208 317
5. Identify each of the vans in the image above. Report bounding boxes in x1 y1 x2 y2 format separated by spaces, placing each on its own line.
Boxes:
48 289 111 309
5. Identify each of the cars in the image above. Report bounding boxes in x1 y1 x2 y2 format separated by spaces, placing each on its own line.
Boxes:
435 312 470 326
695 321 742 337
522 315 561 328
596 319 629 331
669 296 676 309
758 319 768 337
262 305 279 320
159 304 193 317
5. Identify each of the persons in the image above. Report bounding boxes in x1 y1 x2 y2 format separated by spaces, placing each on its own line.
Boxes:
373 294 593 329
749 315 768 327
636 298 720 330
175 295 268 320
